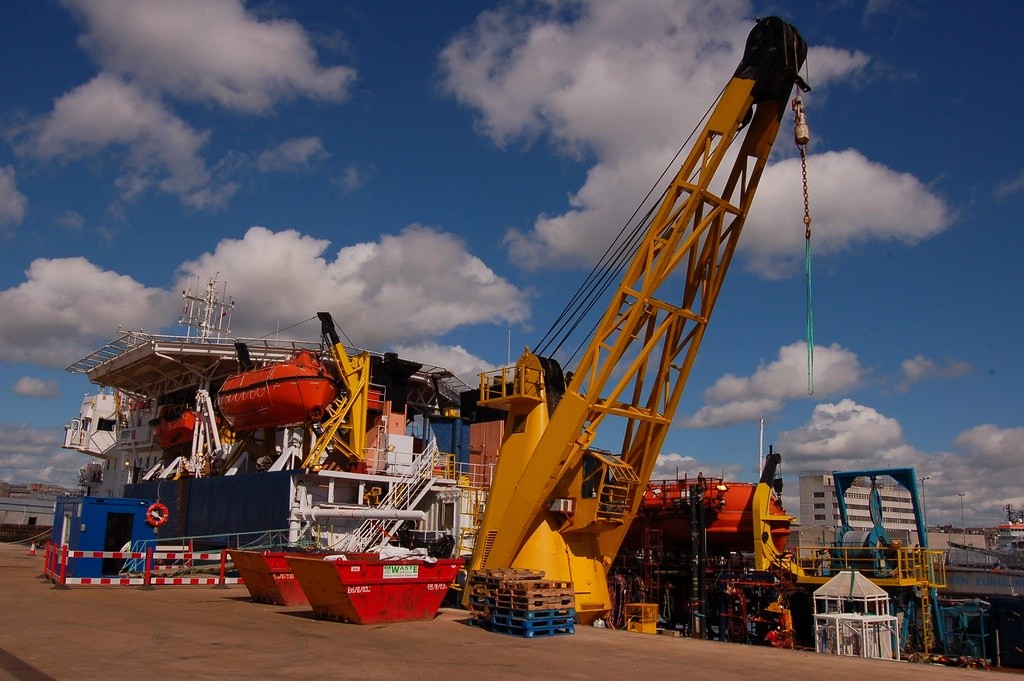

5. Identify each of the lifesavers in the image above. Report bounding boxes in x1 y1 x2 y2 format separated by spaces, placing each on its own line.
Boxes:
146 503 169 526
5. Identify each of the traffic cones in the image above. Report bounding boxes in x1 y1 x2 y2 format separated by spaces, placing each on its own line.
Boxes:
27 540 37 556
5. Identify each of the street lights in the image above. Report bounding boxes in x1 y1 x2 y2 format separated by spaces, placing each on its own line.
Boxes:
959 493 966 544
915 477 931 549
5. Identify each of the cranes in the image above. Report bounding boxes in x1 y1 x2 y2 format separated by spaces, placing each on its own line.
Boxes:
451 16 813 629
